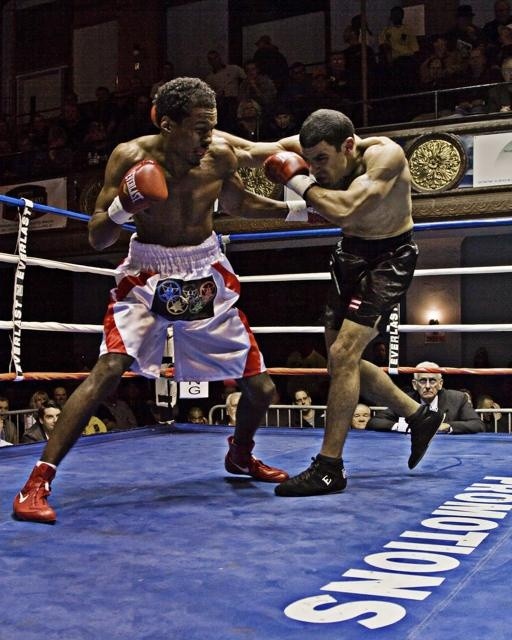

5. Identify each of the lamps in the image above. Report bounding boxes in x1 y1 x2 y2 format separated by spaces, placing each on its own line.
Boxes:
429 319 439 336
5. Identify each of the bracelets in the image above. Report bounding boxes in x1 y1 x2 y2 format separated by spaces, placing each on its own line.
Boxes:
285 200 309 223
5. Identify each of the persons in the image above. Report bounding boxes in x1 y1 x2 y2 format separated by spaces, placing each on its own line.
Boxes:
13 77 329 522
152 107 446 497
1 340 511 452
1 0 512 176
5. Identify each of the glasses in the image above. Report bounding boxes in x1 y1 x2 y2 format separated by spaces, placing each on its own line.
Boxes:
414 379 443 387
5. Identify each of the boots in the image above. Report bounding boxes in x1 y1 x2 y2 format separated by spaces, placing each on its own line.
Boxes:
225 436 290 483
12 463 57 523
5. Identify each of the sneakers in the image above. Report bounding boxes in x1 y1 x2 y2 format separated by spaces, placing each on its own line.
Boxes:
405 403 446 470
275 458 347 496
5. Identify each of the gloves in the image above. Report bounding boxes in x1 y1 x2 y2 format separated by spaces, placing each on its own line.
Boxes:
283 201 332 224
108 158 169 226
263 151 320 200
